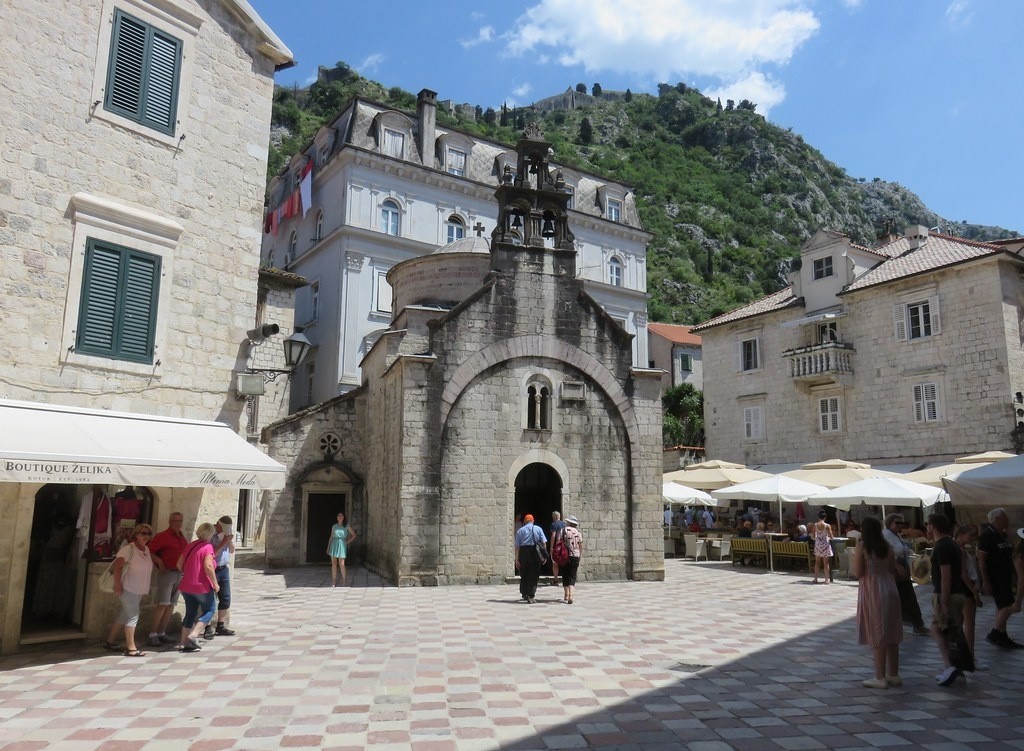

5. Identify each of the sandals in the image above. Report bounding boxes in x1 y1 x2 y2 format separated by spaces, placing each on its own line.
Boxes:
103 641 128 652
124 647 146 656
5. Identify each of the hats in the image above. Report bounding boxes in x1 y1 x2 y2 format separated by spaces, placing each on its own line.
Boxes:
565 515 578 524
218 520 232 535
524 514 534 522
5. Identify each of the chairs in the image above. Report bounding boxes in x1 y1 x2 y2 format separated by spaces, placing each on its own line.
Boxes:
664 529 734 562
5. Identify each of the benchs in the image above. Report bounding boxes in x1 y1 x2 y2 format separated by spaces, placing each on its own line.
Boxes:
730 536 816 574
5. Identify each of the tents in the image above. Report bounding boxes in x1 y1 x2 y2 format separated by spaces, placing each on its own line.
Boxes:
663 452 1024 540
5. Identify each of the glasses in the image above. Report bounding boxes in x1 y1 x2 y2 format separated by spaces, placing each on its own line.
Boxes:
892 520 904 526
138 531 152 537
924 522 931 526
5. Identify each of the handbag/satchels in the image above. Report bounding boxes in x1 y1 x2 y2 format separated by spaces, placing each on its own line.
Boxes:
941 618 975 673
552 541 568 565
532 542 549 562
98 544 133 593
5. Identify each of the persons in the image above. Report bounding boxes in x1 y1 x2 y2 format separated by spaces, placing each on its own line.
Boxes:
148 512 189 646
515 511 583 604
664 505 841 572
812 511 834 584
928 513 976 687
327 512 356 588
76 483 147 561
853 518 904 689
103 524 153 656
177 523 220 651
840 508 1024 672
204 517 237 640
34 518 70 622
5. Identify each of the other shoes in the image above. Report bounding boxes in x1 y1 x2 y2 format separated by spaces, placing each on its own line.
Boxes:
522 593 528 600
204 628 214 640
188 634 201 649
149 636 162 647
527 595 537 603
178 646 195 653
216 625 235 635
856 615 1024 688
568 598 573 604
158 636 177 643
564 596 569 600
811 578 830 584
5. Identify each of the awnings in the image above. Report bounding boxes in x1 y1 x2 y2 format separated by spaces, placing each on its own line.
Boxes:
1 397 287 492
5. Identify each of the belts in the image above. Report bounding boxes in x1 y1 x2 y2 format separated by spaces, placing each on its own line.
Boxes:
216 565 226 569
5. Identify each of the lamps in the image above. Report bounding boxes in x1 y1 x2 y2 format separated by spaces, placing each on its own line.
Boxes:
247 326 312 380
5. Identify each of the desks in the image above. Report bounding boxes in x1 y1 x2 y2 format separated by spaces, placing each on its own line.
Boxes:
829 537 851 566
696 537 723 558
